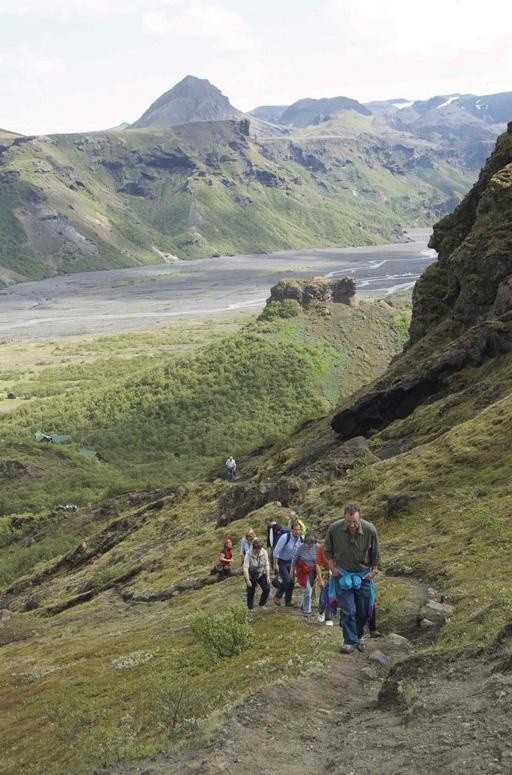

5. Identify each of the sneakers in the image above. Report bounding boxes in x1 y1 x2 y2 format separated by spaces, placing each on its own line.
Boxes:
248 596 380 653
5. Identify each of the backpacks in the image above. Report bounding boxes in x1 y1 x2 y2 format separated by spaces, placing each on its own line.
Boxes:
274 528 291 548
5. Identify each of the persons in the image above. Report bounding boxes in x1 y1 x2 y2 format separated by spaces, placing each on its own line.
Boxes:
325 504 379 652
219 539 233 577
226 455 236 480
369 605 384 638
241 517 338 626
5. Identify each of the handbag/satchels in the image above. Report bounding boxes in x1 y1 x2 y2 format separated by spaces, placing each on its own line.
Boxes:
251 570 259 581
